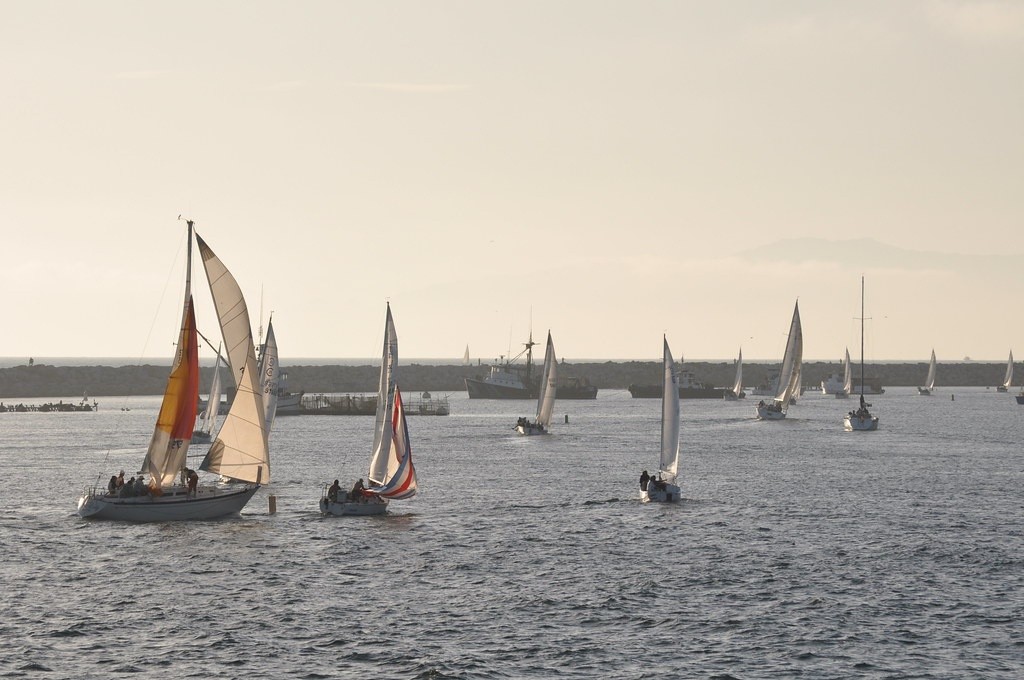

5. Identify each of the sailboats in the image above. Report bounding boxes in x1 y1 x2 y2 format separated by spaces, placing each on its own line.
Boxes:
835 347 852 399
996 349 1014 392
639 332 683 503
512 328 558 437
77 220 280 525
919 350 936 396
722 348 743 401
844 277 879 431
319 302 418 515
197 284 303 415
755 299 804 420
1015 382 1024 405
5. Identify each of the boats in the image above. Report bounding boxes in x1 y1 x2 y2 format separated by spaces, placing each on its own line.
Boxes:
422 390 431 399
464 307 599 400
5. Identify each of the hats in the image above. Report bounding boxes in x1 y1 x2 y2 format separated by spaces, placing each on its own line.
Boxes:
119 470 124 474
359 478 364 482
139 475 144 480
182 467 188 471
334 479 339 483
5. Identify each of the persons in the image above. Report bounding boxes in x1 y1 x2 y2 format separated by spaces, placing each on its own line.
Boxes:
107 476 118 494
857 407 868 415
134 476 145 487
640 470 657 483
758 399 766 407
517 416 530 423
352 478 367 504
116 470 125 490
127 476 136 486
182 467 199 501
328 479 342 502
